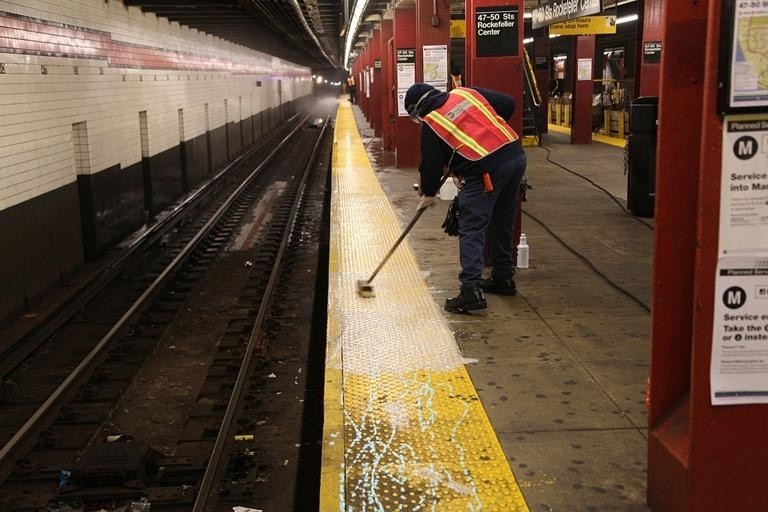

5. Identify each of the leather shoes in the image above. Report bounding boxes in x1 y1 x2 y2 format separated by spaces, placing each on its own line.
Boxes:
445 282 487 311
479 274 516 297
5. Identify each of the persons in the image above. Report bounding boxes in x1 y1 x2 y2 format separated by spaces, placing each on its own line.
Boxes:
346 74 358 104
404 82 527 314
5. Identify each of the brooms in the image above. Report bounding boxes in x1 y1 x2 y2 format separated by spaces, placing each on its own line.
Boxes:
356 168 449 299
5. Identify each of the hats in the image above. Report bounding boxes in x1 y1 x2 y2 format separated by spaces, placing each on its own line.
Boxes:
404 84 440 116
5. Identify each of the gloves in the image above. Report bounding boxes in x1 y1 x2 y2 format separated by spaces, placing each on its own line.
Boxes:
416 194 441 212
441 196 459 236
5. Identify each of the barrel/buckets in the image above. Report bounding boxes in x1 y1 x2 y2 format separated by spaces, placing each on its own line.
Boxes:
440 175 457 201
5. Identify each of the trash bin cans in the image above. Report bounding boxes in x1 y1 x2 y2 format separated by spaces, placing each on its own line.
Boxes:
628 96 658 218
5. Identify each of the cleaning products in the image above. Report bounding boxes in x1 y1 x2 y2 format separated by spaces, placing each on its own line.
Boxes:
517 232 529 269
